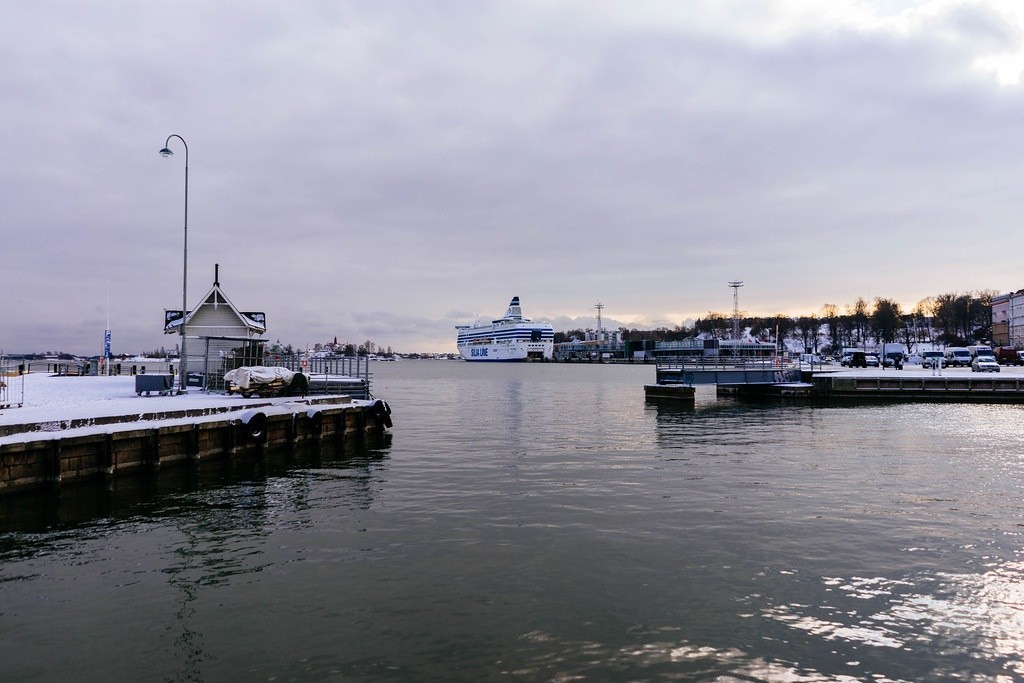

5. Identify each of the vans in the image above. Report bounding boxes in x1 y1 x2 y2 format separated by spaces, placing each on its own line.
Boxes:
944 347 972 367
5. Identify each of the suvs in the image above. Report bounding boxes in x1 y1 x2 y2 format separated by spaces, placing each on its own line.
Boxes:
848 351 867 368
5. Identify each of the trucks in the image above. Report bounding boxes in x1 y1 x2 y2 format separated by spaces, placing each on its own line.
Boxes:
967 346 996 360
800 353 827 365
879 343 905 366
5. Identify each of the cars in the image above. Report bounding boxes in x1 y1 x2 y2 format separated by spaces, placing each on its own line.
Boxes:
921 350 948 367
1017 351 1024 366
971 356 1001 373
865 355 879 367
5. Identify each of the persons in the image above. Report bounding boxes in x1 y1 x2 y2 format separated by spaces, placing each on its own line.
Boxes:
894 353 904 369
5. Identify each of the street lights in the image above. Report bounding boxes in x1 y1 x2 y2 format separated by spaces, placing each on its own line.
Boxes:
158 134 189 394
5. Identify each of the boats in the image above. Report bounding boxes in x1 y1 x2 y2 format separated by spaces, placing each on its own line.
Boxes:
457 296 554 362
